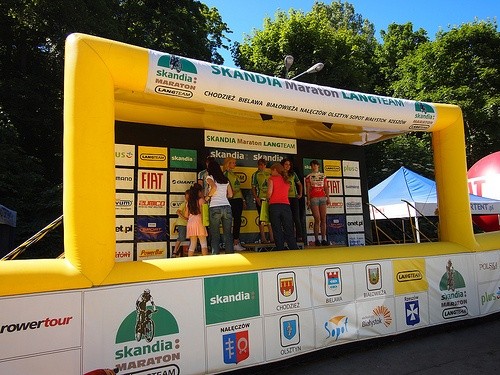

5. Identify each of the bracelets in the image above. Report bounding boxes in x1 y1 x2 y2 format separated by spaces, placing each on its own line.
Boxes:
208 193 212 197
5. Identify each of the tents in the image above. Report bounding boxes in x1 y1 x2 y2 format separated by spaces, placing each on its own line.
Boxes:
368 166 500 244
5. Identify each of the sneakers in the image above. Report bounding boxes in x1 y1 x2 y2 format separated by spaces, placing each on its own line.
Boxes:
233 243 246 251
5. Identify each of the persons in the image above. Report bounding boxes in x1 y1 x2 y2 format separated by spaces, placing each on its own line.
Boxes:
251 158 302 251
305 160 331 246
171 157 246 258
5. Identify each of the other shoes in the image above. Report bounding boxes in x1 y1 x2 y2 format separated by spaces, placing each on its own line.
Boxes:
261 240 275 244
315 239 320 246
321 240 329 246
171 253 176 258
